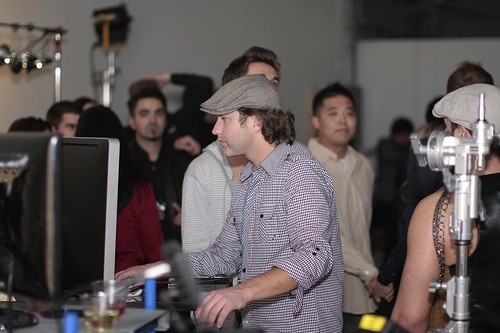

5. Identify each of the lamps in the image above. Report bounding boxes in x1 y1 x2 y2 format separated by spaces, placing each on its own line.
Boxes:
0 23 58 73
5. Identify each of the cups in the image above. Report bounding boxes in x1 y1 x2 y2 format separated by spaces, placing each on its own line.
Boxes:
92 281 126 317
80 294 122 333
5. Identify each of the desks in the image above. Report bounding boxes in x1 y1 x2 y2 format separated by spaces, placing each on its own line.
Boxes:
0 300 173 333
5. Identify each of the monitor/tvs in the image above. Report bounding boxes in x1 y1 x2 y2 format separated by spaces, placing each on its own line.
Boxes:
0 132 120 311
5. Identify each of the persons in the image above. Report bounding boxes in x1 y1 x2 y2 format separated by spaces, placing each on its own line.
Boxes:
113 75 344 333
0 47 500 333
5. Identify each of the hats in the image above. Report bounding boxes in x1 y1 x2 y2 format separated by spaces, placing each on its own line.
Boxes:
432 83 500 136
200 73 282 114
